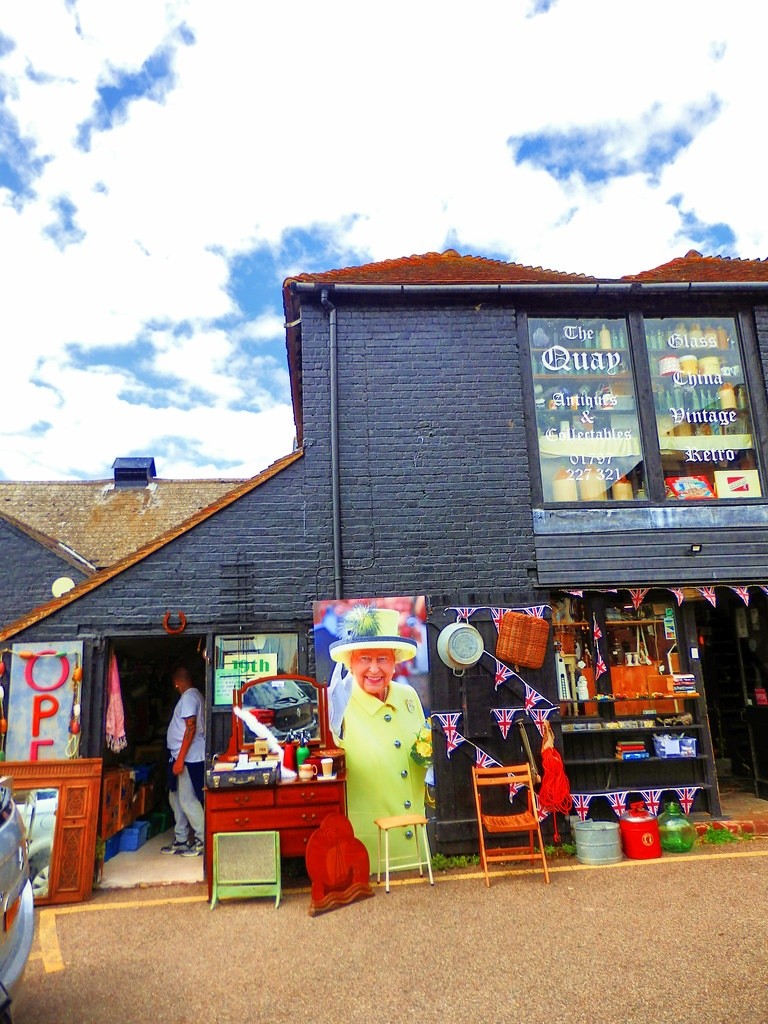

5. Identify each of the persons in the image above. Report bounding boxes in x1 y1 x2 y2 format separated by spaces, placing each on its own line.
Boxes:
160 667 205 856
328 608 433 871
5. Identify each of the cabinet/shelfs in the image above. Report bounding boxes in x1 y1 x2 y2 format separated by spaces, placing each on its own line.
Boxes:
100 762 161 842
533 345 764 501
558 693 721 824
204 748 347 907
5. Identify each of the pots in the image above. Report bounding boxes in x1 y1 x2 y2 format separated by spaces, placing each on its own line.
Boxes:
437 615 484 677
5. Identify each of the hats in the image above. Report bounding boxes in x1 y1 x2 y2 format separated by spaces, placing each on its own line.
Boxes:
329 602 418 664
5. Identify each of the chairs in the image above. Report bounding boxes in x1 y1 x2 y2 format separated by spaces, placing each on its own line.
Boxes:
474 763 551 887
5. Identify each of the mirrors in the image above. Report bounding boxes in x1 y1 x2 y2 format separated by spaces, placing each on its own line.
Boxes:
12 786 60 900
231 676 328 750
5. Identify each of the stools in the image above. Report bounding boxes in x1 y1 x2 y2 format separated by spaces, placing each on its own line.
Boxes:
375 814 434 893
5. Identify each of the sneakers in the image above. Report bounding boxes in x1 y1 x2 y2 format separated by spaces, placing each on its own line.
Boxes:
181 838 205 857
160 837 190 854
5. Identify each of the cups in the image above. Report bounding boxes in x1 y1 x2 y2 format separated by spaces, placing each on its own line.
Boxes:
299 764 317 781
321 758 333 777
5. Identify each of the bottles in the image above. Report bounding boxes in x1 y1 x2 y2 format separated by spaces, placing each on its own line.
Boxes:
285 734 295 770
657 801 695 852
577 676 589 700
297 740 310 772
249 738 279 762
532 325 747 414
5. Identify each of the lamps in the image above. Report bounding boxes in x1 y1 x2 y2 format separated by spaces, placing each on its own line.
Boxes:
684 544 703 555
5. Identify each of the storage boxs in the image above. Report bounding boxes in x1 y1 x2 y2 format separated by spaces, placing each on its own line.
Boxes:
103 831 123 863
151 806 172 833
652 736 697 758
145 814 163 840
120 821 148 851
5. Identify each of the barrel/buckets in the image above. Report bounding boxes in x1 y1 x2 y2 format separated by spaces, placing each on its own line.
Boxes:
575 819 621 863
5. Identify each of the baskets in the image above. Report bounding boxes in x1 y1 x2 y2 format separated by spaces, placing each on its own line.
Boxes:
496 612 550 669
